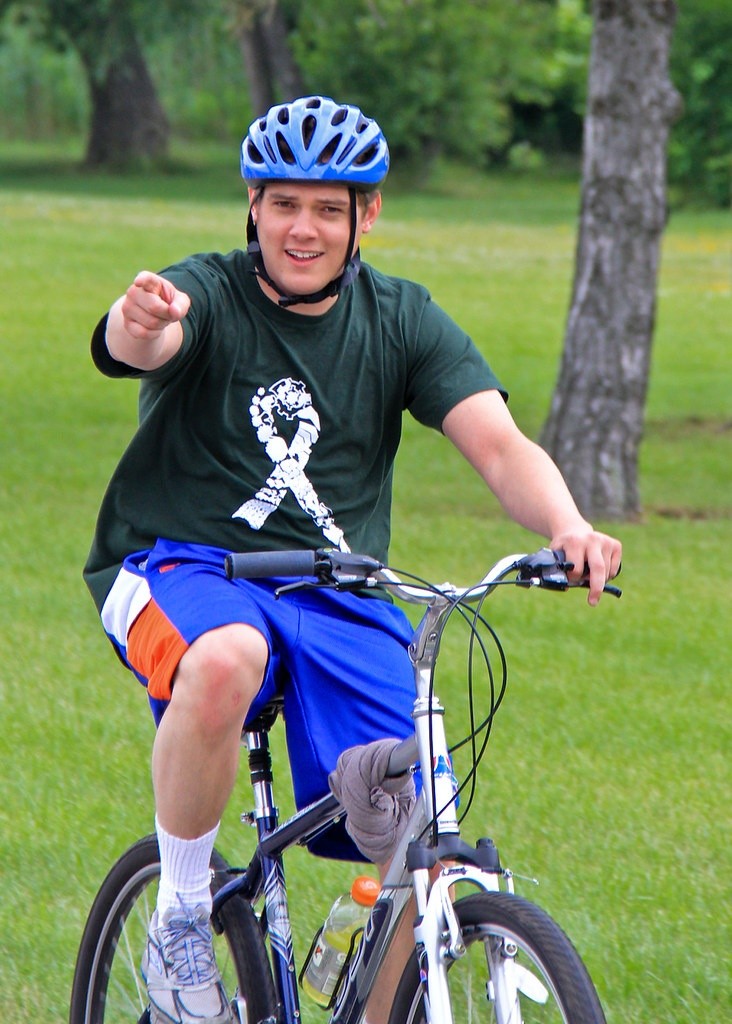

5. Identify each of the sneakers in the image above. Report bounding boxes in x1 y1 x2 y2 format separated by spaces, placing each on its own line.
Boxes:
141 913 237 1024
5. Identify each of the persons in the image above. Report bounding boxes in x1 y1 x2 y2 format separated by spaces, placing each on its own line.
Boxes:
87 92 624 1024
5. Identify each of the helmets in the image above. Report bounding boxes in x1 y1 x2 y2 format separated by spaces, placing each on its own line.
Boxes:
240 96 389 188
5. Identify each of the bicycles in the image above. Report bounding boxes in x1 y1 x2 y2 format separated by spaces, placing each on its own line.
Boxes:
66 548 623 1024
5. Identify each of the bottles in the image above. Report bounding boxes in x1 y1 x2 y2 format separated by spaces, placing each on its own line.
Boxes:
300 874 383 1007
